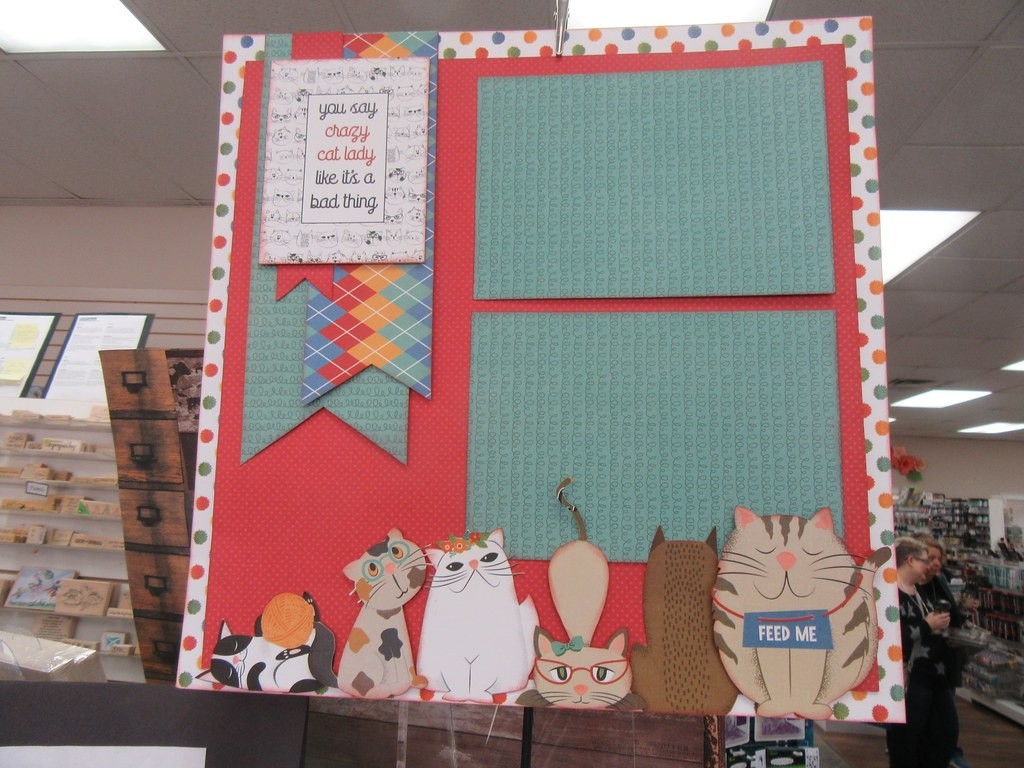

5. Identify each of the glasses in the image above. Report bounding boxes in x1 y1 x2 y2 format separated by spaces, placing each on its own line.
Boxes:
914 556 931 566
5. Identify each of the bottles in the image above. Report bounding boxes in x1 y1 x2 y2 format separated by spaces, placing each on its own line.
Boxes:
934 599 950 634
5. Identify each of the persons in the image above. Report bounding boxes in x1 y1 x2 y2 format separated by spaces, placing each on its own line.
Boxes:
886 533 965 768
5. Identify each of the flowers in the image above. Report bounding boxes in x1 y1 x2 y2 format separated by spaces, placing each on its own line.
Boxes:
890 445 925 480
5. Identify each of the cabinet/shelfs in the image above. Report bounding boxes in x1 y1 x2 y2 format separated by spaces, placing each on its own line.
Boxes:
0 414 140 657
892 487 1024 725
98 349 204 687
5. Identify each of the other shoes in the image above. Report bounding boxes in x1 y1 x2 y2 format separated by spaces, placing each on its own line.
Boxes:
951 750 971 767
